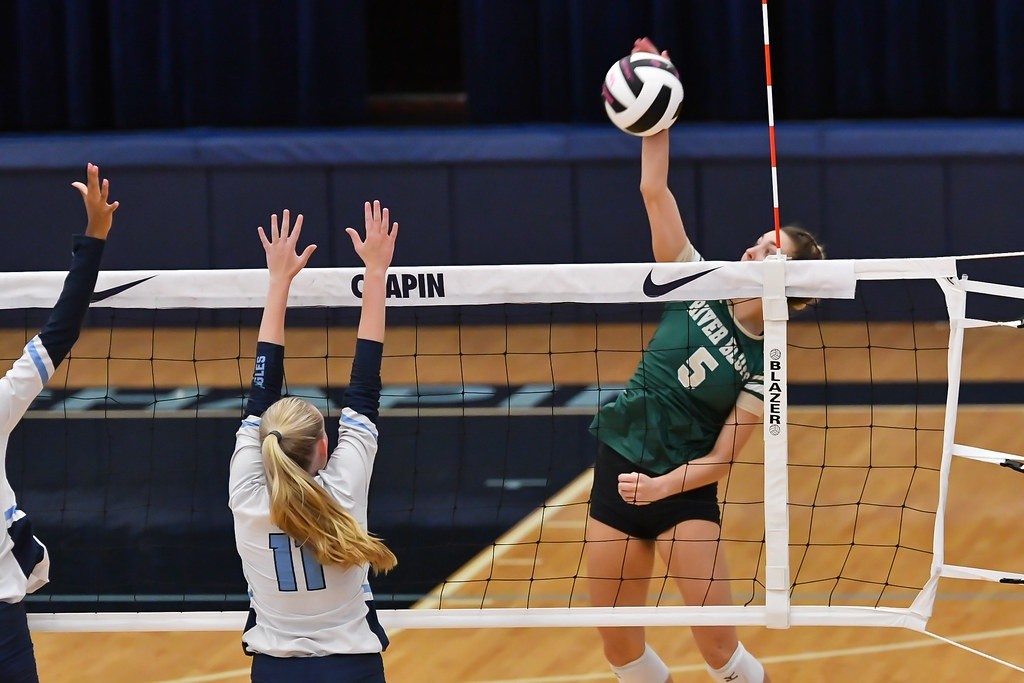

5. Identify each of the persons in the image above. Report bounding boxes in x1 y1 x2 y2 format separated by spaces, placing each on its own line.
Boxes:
582 35 828 682
226 199 398 683
1 162 120 683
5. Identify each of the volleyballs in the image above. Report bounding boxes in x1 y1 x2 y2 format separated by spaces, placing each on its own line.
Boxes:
600 51 686 137
